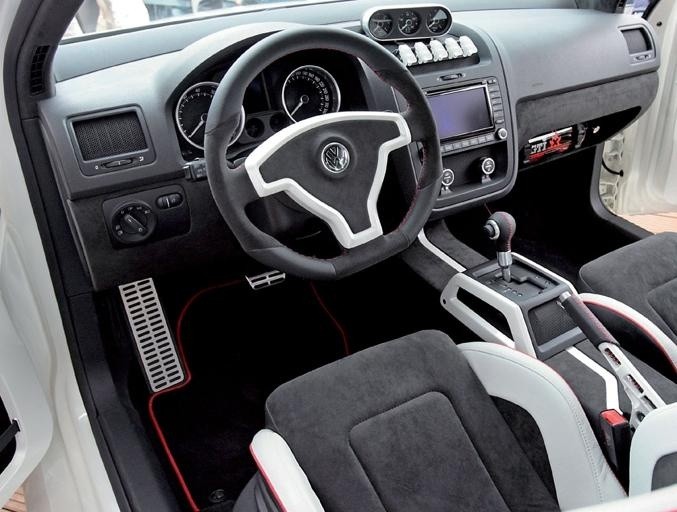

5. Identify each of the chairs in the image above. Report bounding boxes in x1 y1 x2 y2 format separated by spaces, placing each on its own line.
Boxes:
571 230 676 380
256 326 675 512
